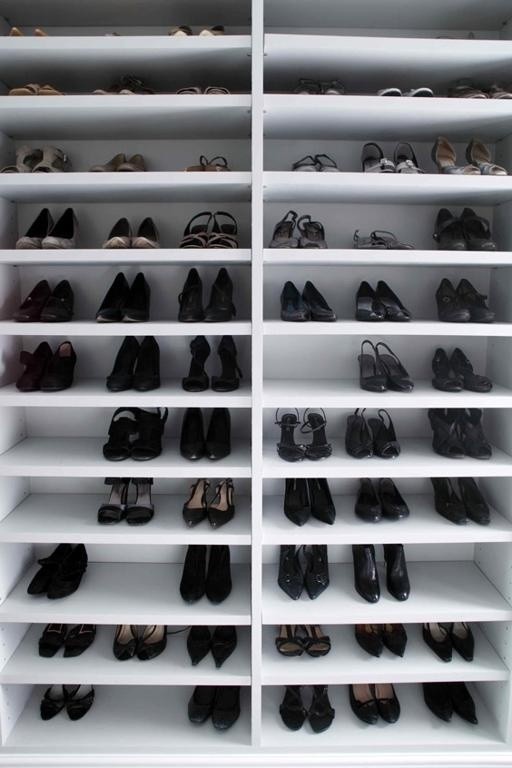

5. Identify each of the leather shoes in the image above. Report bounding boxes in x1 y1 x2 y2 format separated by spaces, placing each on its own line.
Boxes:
27 542 97 721
274 624 477 732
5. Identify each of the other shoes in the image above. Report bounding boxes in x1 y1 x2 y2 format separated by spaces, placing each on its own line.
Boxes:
11 23 246 396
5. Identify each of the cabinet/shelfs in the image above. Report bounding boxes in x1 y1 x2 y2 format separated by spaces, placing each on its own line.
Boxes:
256 3 510 760
1 0 258 752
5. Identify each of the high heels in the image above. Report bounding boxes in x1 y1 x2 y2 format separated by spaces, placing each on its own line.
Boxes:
114 623 168 660
357 340 492 394
276 409 491 529
178 545 242 732
278 545 411 601
99 406 235 531
268 76 511 321
